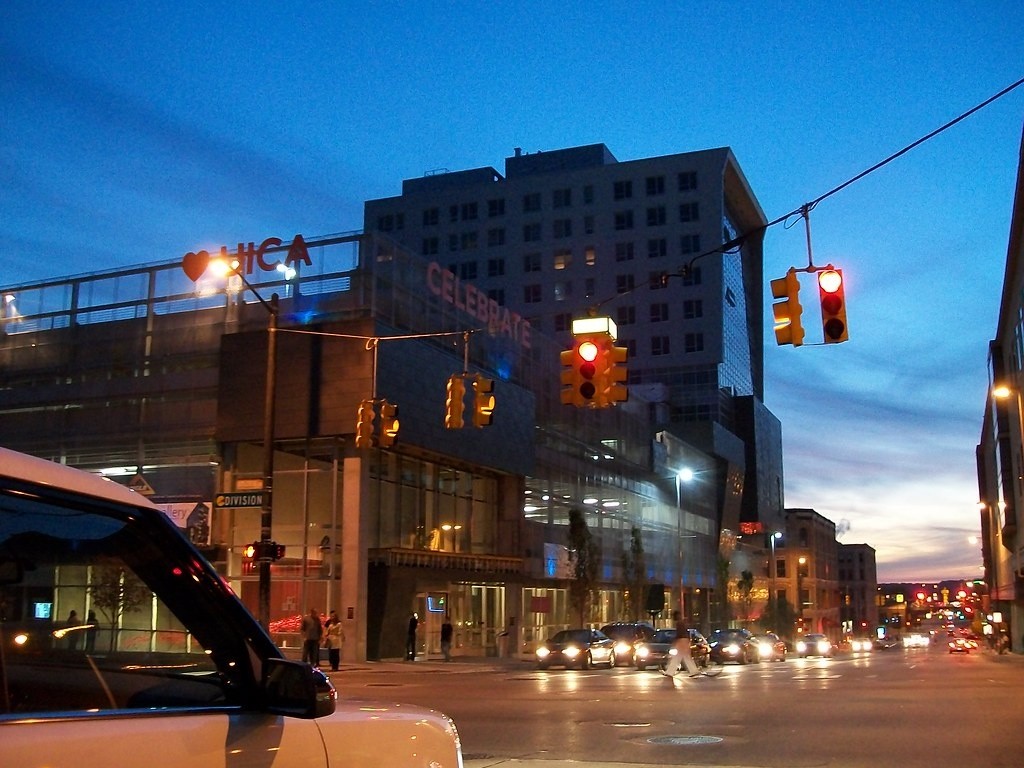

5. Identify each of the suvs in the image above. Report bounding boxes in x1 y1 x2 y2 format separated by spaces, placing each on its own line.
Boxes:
0 448 461 766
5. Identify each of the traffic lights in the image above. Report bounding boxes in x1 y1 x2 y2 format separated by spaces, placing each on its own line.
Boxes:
243 544 255 560
380 404 399 450
817 268 849 344
474 378 494 428
560 335 628 407
769 273 802 347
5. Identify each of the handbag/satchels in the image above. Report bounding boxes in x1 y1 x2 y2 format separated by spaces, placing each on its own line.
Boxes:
324 639 331 651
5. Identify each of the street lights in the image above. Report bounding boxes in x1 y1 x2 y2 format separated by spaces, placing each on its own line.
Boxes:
771 532 782 633
675 466 693 617
994 386 1023 444
211 252 277 633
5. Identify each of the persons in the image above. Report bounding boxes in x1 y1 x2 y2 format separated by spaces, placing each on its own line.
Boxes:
439 616 454 662
67 609 100 652
301 608 345 671
661 611 701 678
404 611 417 662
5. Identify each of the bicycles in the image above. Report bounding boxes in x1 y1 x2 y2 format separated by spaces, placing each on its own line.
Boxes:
659 648 726 678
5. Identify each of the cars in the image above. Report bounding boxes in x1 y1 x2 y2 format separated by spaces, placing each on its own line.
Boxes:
945 620 982 650
601 622 657 667
833 633 930 654
796 634 832 657
949 638 970 654
536 628 616 669
634 628 710 671
707 629 787 664
